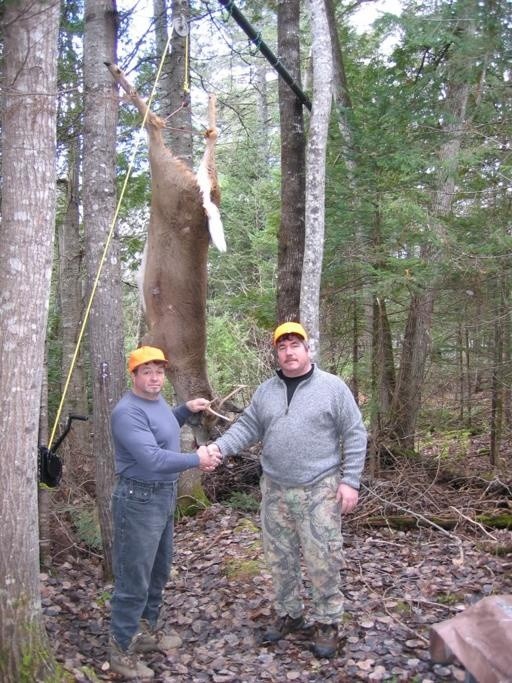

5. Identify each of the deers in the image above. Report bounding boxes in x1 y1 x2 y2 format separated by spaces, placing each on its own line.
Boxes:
103 61 249 450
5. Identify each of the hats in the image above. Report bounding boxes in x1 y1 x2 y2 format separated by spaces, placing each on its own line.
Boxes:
129 346 168 371
273 322 307 346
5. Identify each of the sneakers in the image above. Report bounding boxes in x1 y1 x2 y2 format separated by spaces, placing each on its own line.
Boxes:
264 614 304 641
314 622 337 658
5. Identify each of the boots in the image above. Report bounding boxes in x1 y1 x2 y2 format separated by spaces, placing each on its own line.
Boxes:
110 618 182 677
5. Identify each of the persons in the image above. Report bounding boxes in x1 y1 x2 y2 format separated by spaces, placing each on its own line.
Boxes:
108 346 225 681
200 320 368 659
